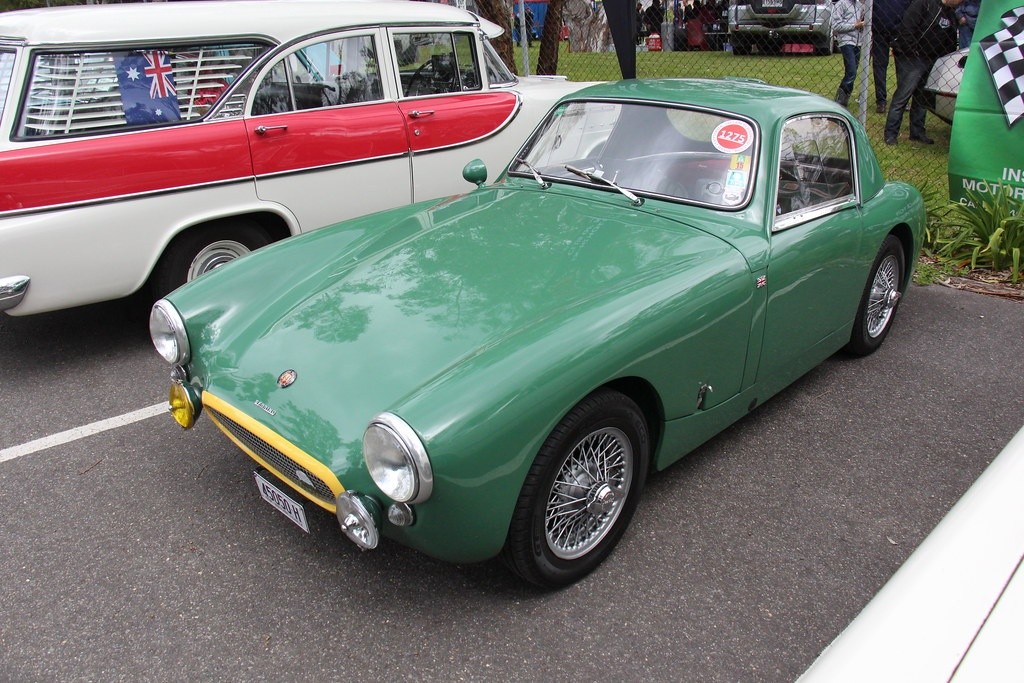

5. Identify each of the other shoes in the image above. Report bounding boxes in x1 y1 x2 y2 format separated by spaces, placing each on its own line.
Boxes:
904 103 911 112
909 132 934 144
884 133 898 146
877 104 886 112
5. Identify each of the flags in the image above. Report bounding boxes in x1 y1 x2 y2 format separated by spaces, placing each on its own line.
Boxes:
111 49 184 127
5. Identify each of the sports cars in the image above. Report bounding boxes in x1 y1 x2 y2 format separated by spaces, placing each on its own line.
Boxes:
145 75 928 590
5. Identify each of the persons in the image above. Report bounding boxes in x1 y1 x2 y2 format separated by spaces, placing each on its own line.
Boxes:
514 10 522 47
955 0 981 50
525 7 535 48
883 0 963 146
831 0 866 108
635 0 729 45
873 0 912 113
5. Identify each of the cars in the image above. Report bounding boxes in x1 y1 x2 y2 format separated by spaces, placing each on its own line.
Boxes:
726 0 842 56
0 5 619 342
